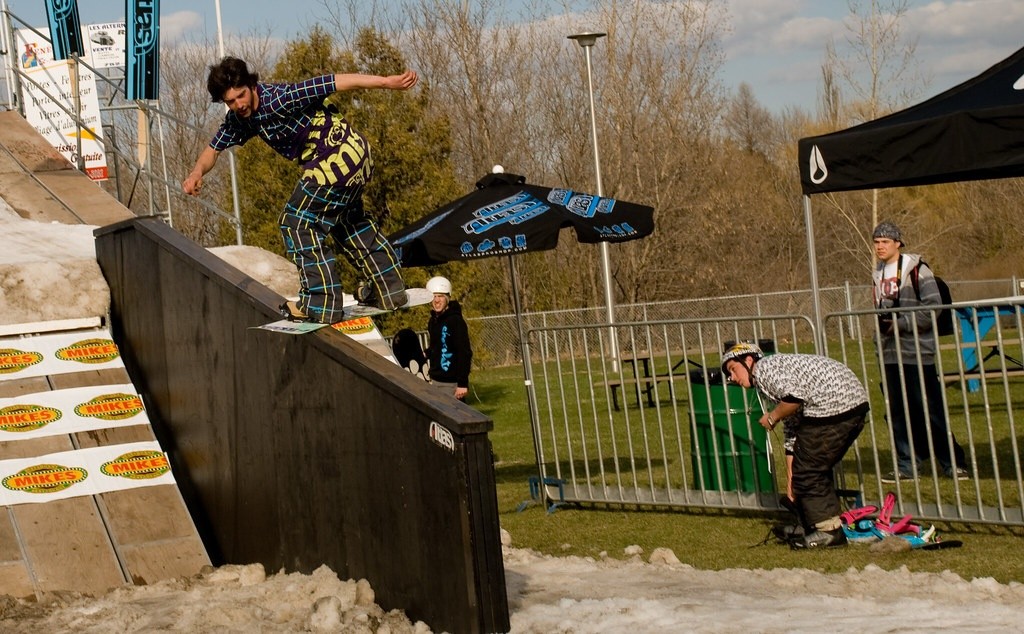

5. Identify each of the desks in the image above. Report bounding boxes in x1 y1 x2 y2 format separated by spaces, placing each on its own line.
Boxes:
615 348 728 407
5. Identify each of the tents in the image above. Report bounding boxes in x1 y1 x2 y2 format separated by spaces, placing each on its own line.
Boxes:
797 46 1024 354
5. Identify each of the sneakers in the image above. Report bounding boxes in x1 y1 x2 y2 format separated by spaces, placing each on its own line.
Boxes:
947 465 969 480
352 284 408 307
789 525 848 551
880 470 921 484
281 300 305 319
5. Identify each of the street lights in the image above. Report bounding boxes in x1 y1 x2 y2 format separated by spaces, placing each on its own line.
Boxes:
568 29 619 373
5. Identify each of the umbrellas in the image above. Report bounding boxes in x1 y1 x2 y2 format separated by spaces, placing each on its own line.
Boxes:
388 166 656 509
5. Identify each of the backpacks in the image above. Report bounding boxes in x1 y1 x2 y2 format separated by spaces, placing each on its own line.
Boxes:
909 260 954 336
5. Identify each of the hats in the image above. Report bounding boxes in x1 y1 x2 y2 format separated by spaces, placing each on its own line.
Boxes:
873 221 905 247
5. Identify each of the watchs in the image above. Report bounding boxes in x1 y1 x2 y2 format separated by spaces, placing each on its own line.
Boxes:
767 414 777 426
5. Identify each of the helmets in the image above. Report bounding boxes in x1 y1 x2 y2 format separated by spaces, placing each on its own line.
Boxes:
721 342 764 375
425 275 452 297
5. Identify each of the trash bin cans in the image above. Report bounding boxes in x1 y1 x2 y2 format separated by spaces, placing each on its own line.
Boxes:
724 340 776 357
687 367 777 493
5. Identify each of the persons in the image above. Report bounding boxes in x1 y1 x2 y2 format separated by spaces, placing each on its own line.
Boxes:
183 57 419 324
873 223 969 483
425 276 472 399
721 343 870 549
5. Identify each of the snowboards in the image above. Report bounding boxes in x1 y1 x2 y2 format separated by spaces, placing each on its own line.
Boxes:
244 287 437 336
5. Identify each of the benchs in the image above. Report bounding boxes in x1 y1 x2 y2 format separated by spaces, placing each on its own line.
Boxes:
594 373 686 411
